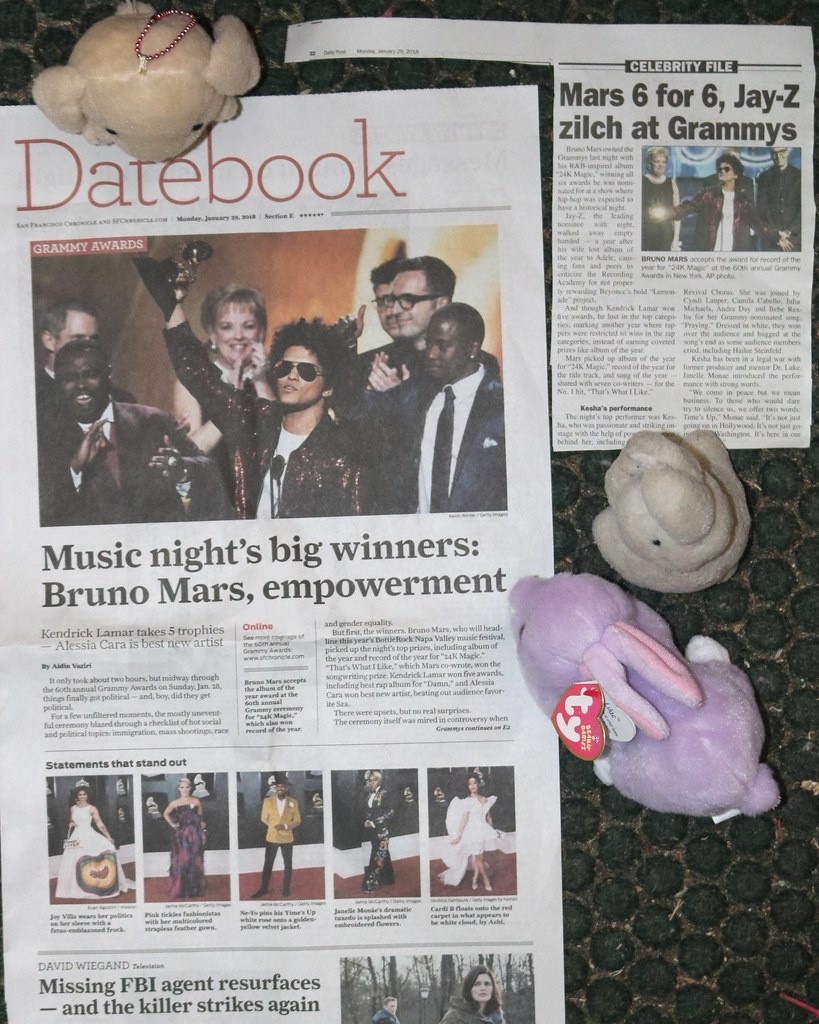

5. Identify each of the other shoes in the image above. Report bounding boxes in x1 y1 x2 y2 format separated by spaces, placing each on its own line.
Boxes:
283 886 292 898
250 887 269 898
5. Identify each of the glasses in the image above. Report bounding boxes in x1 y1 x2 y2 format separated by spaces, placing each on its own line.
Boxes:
396 289 443 313
371 295 397 315
268 353 328 381
770 148 787 157
716 165 735 174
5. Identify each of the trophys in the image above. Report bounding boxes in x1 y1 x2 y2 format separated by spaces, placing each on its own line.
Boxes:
132 241 214 322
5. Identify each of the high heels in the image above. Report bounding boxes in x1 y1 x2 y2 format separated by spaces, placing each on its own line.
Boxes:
469 877 482 892
485 882 495 895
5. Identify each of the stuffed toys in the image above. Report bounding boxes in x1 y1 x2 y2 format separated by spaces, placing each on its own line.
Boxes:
508 572 781 814
591 430 751 591
31 4 261 161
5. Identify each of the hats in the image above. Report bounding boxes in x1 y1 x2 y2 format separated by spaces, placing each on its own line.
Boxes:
271 776 294 789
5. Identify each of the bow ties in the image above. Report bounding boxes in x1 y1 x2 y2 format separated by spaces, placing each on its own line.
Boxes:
370 789 376 794
278 794 286 800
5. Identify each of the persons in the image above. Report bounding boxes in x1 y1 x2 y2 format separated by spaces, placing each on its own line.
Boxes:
361 771 395 893
163 778 208 898
251 775 301 899
55 778 128 900
641 146 801 252
373 996 400 1024
36 255 508 528
439 767 500 892
439 966 504 1024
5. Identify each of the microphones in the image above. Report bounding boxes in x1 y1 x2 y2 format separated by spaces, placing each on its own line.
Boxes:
270 455 285 486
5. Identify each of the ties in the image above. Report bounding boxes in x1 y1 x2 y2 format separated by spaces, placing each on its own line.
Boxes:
430 384 457 512
